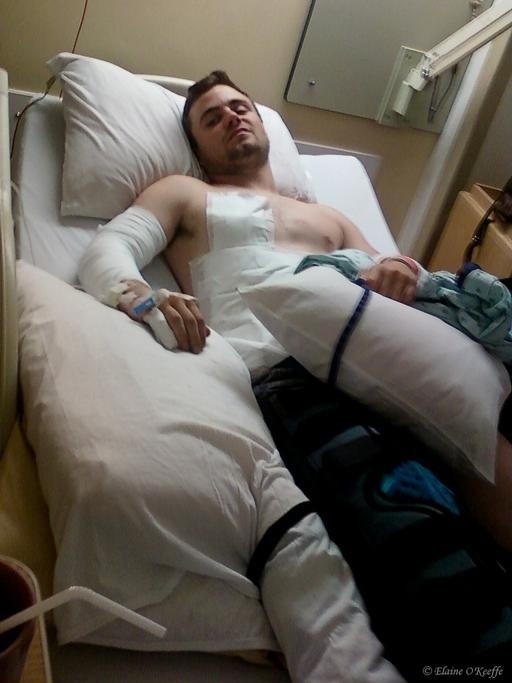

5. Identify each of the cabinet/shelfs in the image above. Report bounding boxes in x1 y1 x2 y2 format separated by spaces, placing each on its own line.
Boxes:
423 180 512 281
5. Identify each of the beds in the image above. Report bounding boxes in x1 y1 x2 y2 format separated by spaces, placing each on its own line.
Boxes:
0 64 402 679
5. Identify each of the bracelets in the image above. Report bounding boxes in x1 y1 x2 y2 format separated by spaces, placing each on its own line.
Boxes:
380 256 417 273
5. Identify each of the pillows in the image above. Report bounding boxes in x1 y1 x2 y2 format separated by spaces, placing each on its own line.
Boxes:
46 51 318 223
10 256 284 652
236 250 511 492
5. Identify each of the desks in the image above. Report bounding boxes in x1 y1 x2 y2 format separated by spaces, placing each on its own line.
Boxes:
0 554 53 681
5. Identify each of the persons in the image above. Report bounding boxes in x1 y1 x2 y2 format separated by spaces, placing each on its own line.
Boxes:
79 66 512 681
